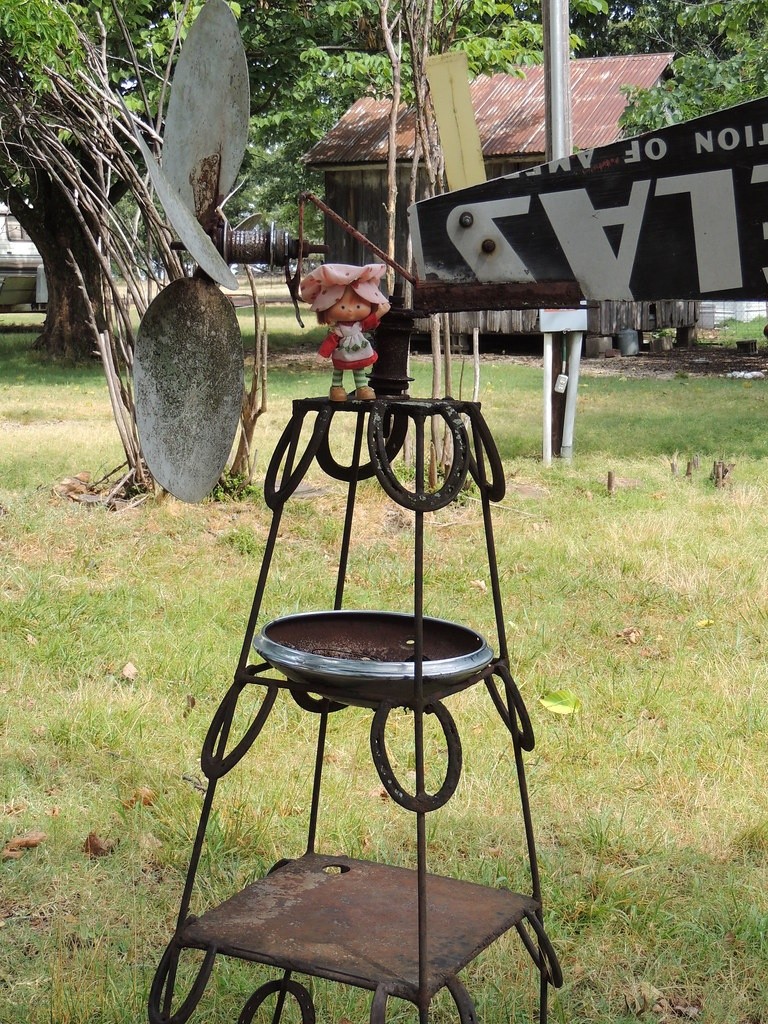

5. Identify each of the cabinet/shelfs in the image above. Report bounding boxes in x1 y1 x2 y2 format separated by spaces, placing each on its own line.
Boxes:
146 280 565 1024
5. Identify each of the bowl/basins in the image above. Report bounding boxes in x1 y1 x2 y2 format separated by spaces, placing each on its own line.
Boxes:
253 610 495 707
